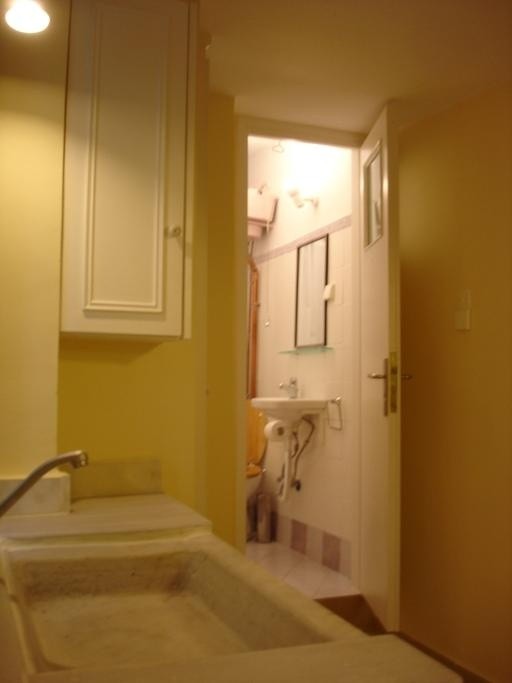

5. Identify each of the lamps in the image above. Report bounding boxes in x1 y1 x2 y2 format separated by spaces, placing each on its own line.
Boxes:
290 189 319 208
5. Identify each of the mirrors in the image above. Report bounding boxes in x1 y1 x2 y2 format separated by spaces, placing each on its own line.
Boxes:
295 235 328 346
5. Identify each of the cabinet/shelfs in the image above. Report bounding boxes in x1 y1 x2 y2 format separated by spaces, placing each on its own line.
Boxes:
61 0 196 340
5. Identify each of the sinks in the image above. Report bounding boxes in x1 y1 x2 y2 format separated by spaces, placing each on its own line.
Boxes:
252 394 329 418
0 534 342 682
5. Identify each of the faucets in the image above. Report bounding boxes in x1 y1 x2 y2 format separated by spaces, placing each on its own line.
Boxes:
1 447 90 515
278 376 300 397
